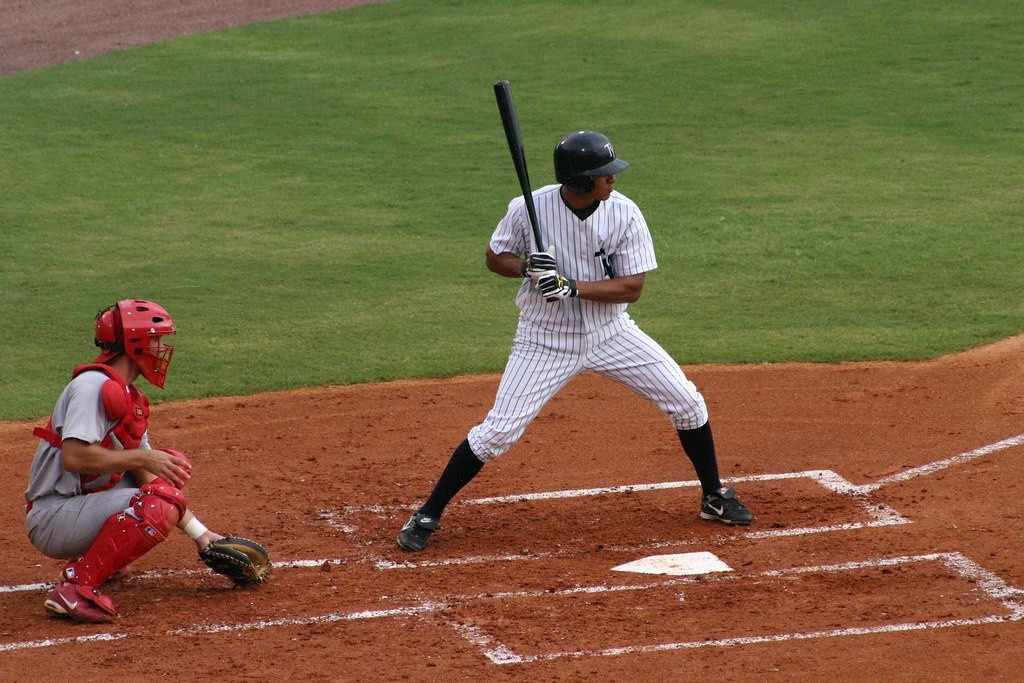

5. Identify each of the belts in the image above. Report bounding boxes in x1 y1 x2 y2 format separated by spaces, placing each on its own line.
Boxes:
25 501 33 515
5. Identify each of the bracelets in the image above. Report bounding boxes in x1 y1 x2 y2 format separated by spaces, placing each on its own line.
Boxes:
181 516 209 539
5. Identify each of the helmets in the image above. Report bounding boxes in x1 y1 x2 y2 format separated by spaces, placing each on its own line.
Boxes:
92 307 123 363
554 131 629 194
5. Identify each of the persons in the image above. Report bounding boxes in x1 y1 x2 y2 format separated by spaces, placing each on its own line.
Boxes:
24 298 225 622
396 130 754 553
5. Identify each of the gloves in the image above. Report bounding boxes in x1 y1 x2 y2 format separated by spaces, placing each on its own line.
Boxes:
520 245 556 277
535 273 578 303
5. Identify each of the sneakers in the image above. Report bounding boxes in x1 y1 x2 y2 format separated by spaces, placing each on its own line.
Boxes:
700 485 752 525
43 580 116 623
397 512 441 552
60 561 130 582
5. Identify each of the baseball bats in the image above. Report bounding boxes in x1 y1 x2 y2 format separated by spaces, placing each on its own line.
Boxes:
490 78 560 304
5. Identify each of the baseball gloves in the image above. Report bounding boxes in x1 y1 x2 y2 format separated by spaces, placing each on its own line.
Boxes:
196 525 272 591
